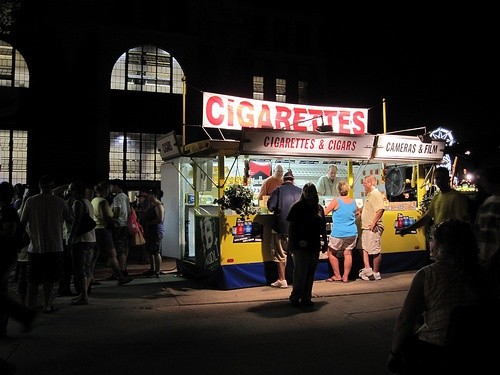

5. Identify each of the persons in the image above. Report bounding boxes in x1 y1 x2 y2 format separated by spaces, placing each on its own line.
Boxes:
259 165 284 200
0 176 162 339
324 181 358 284
359 175 386 280
386 167 500 375
286 183 328 308
317 165 346 196
267 172 303 288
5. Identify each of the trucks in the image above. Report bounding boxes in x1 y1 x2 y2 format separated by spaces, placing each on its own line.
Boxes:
155 126 477 290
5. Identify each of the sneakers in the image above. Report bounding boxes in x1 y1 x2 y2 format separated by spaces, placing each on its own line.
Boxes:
271 279 288 289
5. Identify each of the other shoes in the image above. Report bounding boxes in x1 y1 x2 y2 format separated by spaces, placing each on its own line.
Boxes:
358 268 372 277
143 269 159 278
118 276 134 285
289 298 301 307
70 295 88 305
111 271 128 280
362 272 382 282
301 299 314 306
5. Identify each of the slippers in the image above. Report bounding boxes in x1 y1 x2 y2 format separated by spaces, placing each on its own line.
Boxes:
326 277 342 282
43 306 58 314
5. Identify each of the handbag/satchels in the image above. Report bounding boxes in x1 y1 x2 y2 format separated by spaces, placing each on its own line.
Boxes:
67 198 96 237
127 214 139 236
130 231 147 246
